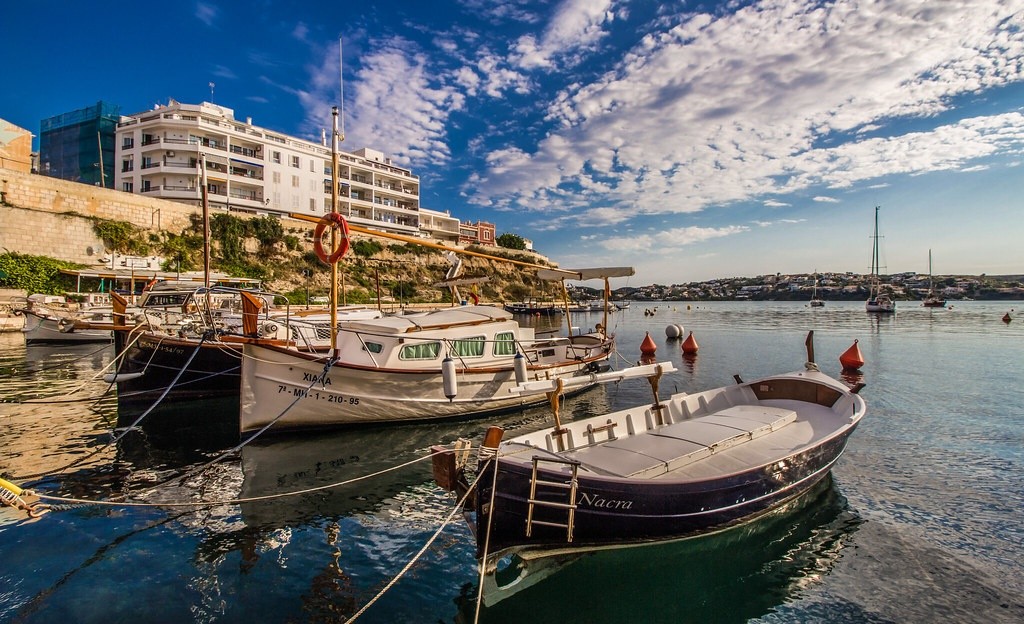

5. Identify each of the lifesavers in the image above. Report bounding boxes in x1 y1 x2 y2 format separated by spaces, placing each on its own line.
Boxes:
313 213 350 264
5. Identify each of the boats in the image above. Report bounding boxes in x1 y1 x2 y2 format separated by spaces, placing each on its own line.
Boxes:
109 141 383 405
13 302 135 347
503 298 632 314
475 331 866 609
219 105 638 440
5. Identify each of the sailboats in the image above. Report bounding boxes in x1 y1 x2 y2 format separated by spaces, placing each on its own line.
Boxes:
810 269 826 307
922 249 946 308
866 208 895 312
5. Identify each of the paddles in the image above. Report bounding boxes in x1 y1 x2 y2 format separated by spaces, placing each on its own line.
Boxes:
508 361 678 401
